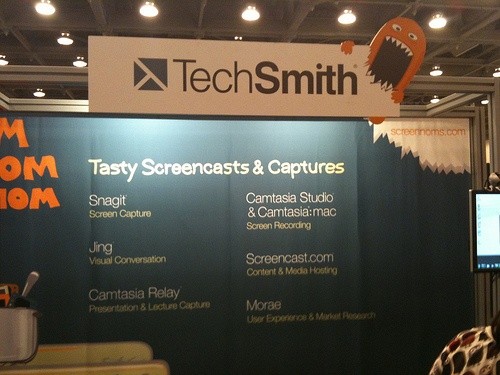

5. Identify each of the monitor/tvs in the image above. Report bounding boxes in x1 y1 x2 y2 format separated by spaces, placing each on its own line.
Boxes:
468 189 500 272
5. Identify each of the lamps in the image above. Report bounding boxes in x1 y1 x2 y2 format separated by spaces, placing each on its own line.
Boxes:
241 0 260 21
0 54 9 65
139 0 158 17
429 66 443 76
430 95 440 103
35 0 55 15
33 87 46 98
57 32 73 45
429 0 447 29
338 0 356 24
493 68 500 78
73 56 87 67
